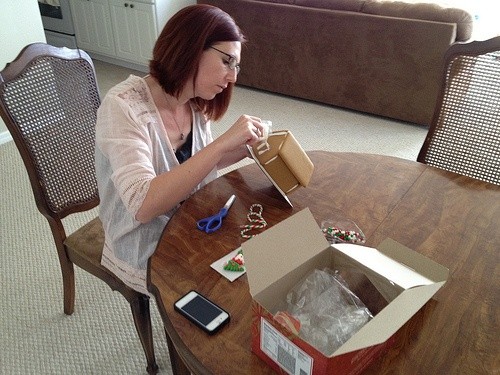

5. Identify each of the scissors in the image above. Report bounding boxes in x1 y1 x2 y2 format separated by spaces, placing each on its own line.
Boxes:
196 194 236 233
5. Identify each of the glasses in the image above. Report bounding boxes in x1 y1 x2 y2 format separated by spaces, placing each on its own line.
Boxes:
209 46 240 74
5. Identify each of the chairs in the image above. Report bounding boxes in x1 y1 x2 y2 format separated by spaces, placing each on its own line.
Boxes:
0 43 158 375
416 34 500 185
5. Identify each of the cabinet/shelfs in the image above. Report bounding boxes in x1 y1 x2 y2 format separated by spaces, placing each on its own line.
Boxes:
69 0 198 75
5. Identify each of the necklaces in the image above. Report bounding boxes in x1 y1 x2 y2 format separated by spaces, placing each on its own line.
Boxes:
162 91 184 140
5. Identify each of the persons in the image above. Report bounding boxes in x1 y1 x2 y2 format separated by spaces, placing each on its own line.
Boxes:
94 3 264 299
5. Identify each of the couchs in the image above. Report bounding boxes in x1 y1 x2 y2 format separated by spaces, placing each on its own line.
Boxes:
197 0 473 127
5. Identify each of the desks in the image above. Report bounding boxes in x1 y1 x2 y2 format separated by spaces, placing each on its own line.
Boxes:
147 151 500 375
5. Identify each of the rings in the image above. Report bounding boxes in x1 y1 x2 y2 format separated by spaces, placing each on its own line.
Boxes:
255 128 259 134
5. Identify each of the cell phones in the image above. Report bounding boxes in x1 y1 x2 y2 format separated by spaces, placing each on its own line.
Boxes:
174 290 230 336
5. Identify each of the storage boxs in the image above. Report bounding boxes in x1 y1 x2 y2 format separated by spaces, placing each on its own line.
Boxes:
240 207 451 375
